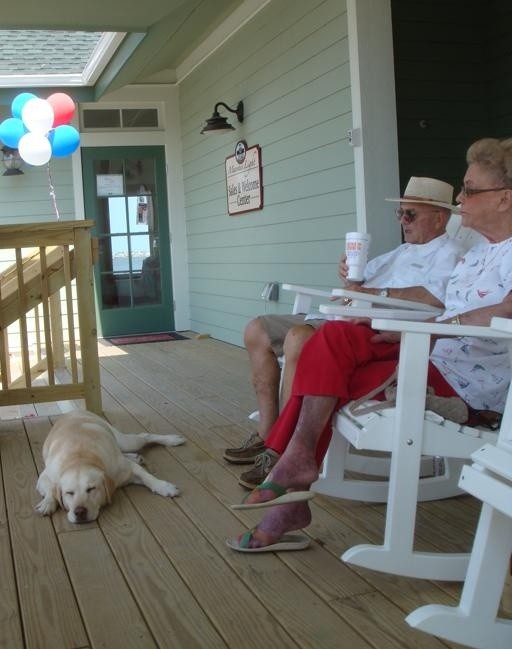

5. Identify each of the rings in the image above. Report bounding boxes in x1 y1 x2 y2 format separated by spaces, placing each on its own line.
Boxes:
343 297 351 305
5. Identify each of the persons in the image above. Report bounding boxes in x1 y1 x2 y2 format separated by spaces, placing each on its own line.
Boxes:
222 177 468 491
227 139 510 552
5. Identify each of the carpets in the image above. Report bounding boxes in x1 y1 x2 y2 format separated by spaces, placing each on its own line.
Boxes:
96 332 193 348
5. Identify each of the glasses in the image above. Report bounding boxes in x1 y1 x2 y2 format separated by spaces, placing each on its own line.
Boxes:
465 186 511 200
394 208 442 223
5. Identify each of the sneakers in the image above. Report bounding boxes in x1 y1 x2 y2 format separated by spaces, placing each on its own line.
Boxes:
239 447 280 489
222 431 267 464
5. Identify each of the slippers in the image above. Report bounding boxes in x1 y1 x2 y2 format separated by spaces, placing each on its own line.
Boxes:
229 481 317 509
225 524 310 553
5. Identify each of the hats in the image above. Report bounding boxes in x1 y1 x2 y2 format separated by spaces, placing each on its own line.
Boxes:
384 176 463 216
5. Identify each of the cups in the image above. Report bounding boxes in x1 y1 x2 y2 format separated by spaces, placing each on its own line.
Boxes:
344 232 372 283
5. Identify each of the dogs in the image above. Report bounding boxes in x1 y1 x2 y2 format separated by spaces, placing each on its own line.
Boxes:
34 409 188 524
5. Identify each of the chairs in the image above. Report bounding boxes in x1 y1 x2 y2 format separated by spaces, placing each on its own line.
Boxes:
306 289 510 585
249 277 478 506
404 372 511 648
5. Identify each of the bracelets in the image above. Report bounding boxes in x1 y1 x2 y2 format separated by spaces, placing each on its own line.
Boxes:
451 315 463 340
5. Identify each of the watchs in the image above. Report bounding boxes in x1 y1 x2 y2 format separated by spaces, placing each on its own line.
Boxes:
379 288 390 299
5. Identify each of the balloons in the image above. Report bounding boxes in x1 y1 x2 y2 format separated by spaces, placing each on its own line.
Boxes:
1 91 81 166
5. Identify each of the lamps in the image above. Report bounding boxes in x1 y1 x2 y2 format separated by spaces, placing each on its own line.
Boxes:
1 144 28 179
197 99 246 138
